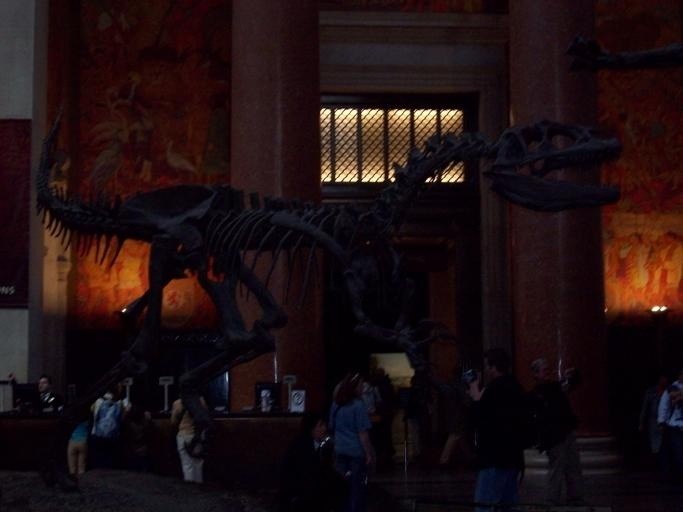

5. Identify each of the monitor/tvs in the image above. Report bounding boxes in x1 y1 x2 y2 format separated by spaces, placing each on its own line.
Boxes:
15 382 40 404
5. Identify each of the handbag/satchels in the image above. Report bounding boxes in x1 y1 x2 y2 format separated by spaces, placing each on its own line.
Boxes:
318 406 342 465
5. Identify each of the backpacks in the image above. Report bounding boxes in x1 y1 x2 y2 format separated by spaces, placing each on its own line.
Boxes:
183 435 202 460
